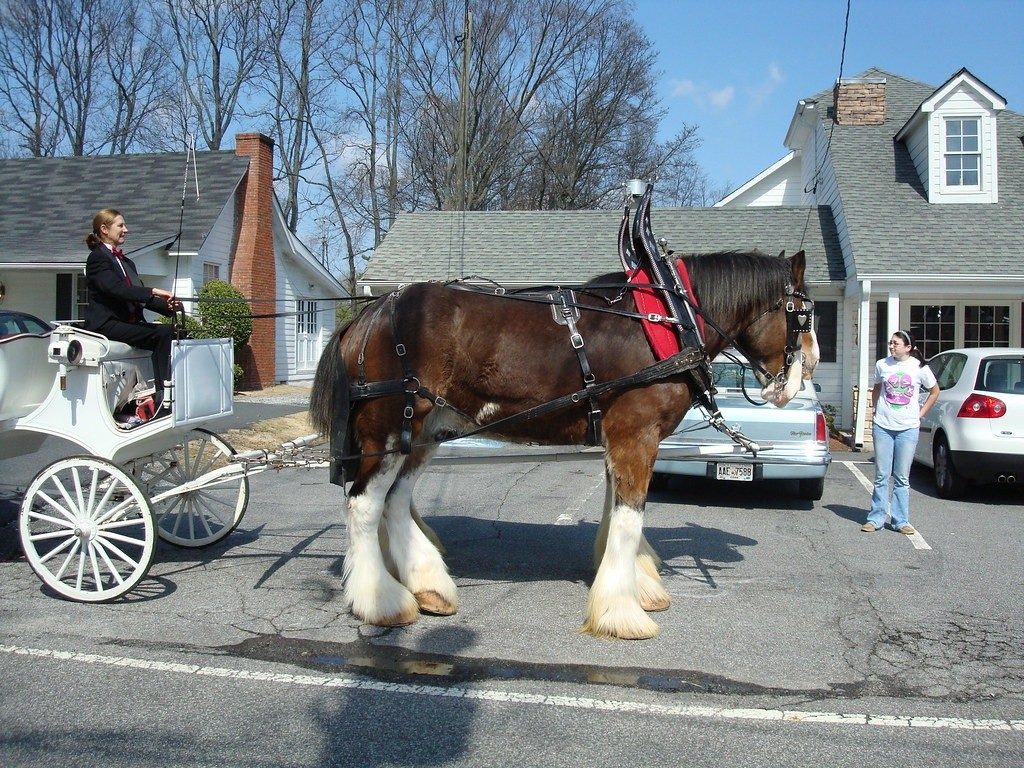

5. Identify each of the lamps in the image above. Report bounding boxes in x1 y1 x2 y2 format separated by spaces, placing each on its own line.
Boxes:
0 280 6 300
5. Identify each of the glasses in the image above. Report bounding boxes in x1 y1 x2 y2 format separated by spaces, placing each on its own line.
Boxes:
888 342 906 347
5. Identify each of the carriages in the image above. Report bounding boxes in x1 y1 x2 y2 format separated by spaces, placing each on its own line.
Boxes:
0 163 821 639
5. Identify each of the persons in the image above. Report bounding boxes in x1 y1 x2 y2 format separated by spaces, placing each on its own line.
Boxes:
860 330 940 535
83 208 182 407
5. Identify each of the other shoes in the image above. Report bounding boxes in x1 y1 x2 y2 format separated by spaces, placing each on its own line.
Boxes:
898 526 914 534
861 523 875 531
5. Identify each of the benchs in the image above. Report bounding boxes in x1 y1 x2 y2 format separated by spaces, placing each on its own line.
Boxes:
49 319 156 402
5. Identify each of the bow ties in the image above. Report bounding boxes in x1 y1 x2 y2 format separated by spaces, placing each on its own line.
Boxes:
112 246 123 260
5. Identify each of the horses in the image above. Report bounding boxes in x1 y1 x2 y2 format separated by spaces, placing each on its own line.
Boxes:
304 245 821 642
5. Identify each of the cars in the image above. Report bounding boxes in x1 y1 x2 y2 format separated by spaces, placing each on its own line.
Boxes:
650 346 830 498
915 348 1024 494
